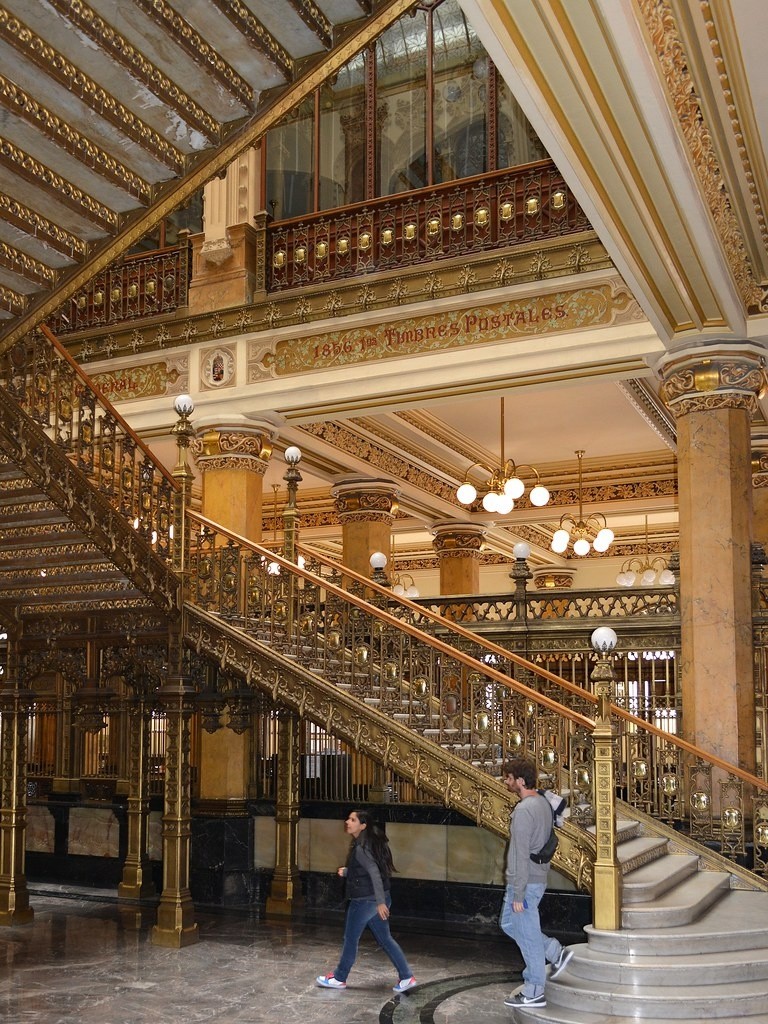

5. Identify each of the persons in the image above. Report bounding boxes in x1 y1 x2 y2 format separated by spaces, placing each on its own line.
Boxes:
501 757 575 1007
317 810 417 992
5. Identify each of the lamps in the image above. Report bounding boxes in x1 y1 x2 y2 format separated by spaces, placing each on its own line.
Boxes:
455 396 551 515
282 445 301 516
391 535 419 598
616 513 676 587
170 394 197 479
509 541 534 626
134 517 176 545
368 552 390 614
258 484 306 577
551 449 615 557
589 626 620 739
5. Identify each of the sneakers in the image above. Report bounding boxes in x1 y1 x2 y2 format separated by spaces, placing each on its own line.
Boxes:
316 972 347 988
549 946 574 978
393 976 417 992
504 990 546 1007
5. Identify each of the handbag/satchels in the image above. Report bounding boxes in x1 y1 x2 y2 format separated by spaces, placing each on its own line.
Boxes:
529 792 559 865
